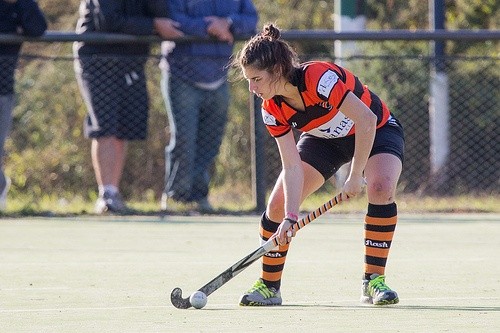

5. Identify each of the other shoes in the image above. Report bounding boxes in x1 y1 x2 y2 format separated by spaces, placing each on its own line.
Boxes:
96 193 127 214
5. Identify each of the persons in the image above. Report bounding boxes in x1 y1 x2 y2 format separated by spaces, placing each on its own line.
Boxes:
161 0 258 210
75 0 184 212
0 0 48 203
240 24 406 305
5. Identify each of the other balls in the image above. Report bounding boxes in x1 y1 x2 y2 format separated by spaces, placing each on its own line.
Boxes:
189 291 207 309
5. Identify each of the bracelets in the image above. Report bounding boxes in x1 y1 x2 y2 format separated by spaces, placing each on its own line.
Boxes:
283 218 297 224
285 212 298 221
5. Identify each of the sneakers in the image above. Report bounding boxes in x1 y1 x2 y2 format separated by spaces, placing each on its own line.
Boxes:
360 275 398 306
240 280 282 306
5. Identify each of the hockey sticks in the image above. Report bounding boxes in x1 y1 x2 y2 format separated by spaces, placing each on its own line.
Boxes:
170 191 353 313
